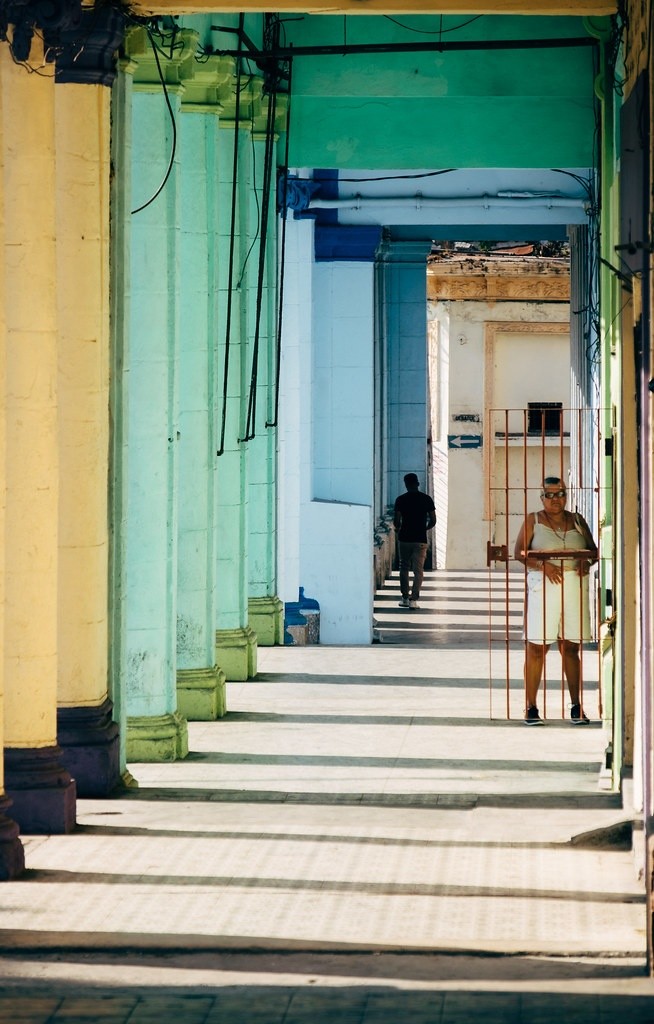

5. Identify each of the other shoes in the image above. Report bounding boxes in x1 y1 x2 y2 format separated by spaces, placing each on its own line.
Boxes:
409 600 420 609
399 597 409 607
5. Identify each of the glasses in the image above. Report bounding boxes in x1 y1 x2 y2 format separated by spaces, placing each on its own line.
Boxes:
541 491 566 499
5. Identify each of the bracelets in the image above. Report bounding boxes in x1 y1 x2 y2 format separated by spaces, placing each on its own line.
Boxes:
537 561 543 567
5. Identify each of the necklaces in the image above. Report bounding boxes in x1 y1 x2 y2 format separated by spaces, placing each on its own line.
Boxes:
543 510 567 549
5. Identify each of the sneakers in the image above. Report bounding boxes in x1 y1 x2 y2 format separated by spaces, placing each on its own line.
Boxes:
524 704 544 725
568 703 589 725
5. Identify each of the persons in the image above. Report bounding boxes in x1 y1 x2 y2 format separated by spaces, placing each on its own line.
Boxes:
393 473 436 609
515 477 598 726
